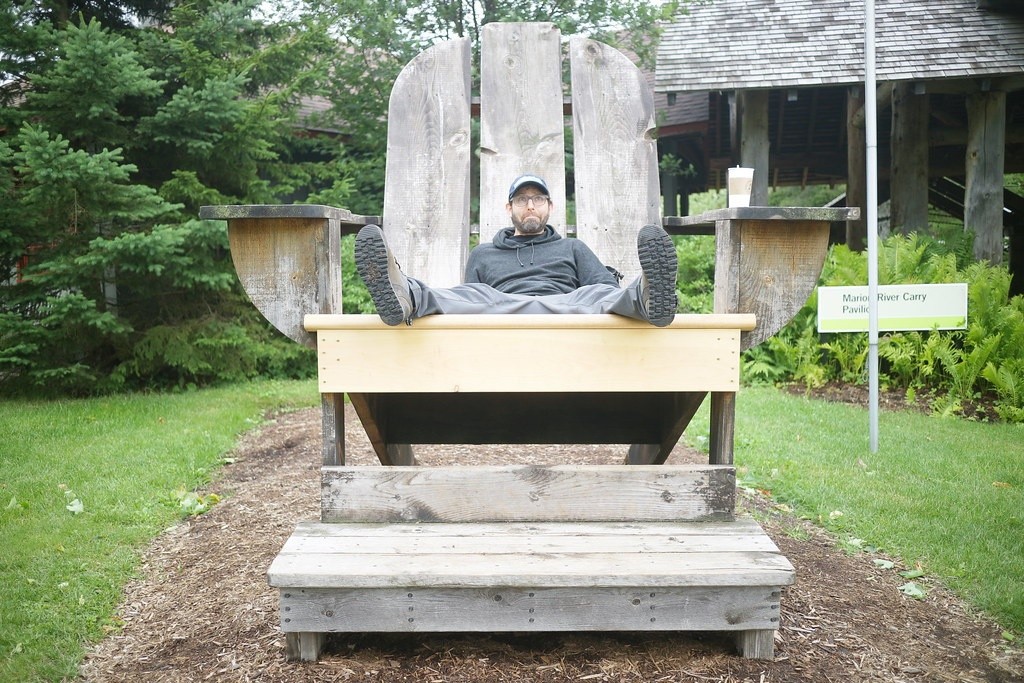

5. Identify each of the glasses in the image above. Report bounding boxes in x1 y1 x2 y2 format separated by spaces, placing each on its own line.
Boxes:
511 193 548 208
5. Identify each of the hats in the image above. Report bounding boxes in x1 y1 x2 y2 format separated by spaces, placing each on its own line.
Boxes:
508 175 550 201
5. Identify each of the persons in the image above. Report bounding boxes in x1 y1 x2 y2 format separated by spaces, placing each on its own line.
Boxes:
354 174 677 327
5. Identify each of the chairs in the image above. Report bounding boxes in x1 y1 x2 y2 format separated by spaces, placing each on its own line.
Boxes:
204 18 862 666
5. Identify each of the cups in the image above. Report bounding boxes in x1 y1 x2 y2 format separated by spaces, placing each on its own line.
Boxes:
728 168 754 208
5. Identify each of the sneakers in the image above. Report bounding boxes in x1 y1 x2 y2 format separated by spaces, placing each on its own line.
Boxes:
637 225 678 327
354 224 414 327
